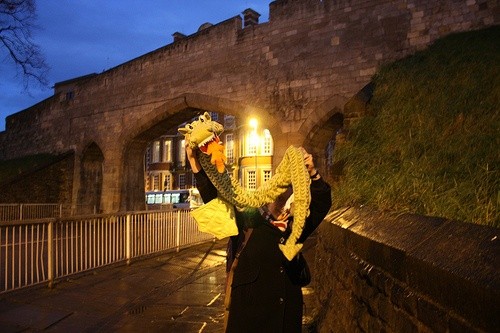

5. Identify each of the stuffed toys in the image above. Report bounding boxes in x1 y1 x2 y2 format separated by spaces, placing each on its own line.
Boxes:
178 110 311 261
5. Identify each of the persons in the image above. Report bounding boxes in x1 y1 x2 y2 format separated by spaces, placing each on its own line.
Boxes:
183 130 333 332
